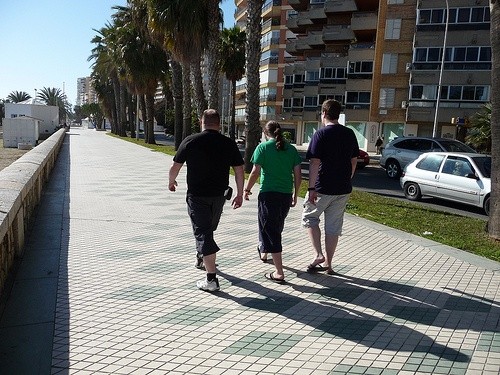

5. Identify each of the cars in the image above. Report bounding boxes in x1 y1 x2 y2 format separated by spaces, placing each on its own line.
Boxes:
378 137 478 179
400 151 491 216
297 151 365 173
357 149 370 169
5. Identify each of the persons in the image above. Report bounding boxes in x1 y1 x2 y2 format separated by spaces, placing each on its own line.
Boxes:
374 136 384 154
243 121 302 283
302 100 359 274
168 109 244 291
452 161 464 176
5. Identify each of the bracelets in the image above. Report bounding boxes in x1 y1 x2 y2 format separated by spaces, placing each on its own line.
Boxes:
307 188 315 191
244 188 250 192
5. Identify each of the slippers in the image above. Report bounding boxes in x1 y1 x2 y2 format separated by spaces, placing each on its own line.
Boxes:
264 271 285 284
257 244 267 261
323 264 333 274
307 263 329 271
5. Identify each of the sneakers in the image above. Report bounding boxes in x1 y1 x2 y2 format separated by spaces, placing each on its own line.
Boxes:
197 277 221 293
194 253 205 268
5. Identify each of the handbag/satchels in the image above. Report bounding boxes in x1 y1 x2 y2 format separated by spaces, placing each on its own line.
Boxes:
224 186 234 201
